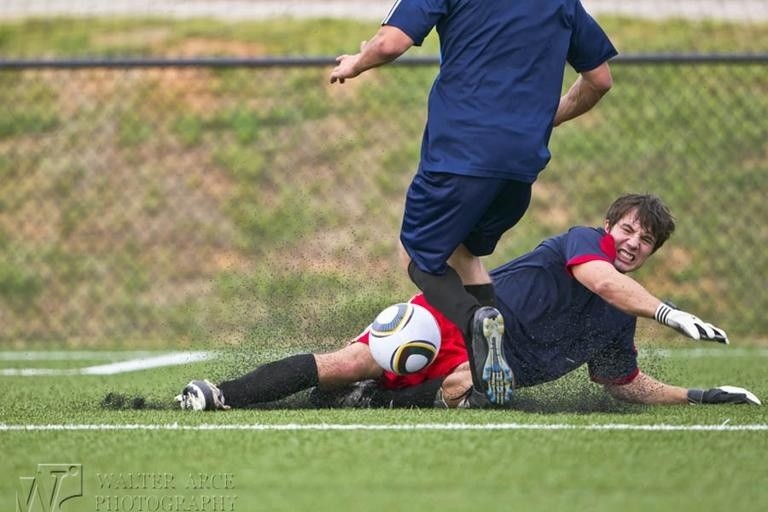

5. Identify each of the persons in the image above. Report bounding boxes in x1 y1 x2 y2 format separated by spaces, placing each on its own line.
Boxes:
330 0 619 408
172 193 762 412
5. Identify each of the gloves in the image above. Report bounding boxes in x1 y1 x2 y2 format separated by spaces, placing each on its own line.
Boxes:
655 298 730 344
687 385 761 406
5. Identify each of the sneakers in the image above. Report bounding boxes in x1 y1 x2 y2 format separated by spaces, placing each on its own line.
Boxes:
175 379 231 411
470 306 515 408
310 379 380 407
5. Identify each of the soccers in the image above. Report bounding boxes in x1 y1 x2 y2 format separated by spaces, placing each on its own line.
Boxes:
369 303 441 375
